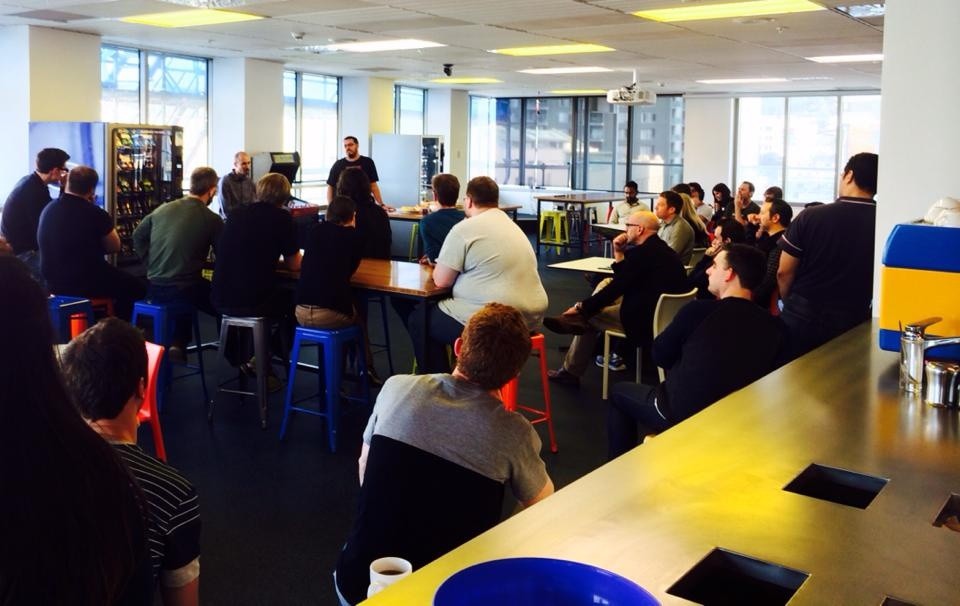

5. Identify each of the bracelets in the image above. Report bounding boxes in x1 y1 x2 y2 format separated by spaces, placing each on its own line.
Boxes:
380 204 385 207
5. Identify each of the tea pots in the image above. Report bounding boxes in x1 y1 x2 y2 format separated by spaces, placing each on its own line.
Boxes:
924 197 960 223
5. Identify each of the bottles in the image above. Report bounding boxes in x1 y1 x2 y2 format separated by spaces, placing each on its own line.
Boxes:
70 312 87 339
925 361 960 408
899 325 924 393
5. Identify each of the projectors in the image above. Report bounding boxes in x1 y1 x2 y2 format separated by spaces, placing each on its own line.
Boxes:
606 89 658 106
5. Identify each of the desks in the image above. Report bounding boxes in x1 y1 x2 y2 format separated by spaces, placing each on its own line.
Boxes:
273 257 451 373
533 192 659 260
388 205 522 261
546 258 694 275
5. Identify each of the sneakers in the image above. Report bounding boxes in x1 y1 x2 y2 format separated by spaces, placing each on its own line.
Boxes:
596 354 626 370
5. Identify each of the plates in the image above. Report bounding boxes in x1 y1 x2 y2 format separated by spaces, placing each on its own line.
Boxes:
431 557 663 606
395 208 422 213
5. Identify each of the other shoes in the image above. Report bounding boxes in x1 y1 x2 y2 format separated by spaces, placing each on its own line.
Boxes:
264 374 280 392
169 347 188 365
240 356 258 376
543 314 584 335
549 366 576 385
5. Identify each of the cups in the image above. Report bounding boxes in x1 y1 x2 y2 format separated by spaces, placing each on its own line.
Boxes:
933 210 960 226
366 557 412 598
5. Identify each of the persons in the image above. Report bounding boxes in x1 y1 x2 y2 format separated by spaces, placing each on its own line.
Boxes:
131 168 249 370
543 152 879 395
290 195 384 398
313 168 393 311
772 153 878 357
333 303 553 606
326 136 395 216
218 152 257 219
210 173 314 394
595 242 803 464
0 147 141 325
390 174 470 332
407 176 549 375
0 233 204 605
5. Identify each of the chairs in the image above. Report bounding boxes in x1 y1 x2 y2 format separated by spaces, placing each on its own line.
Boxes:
139 340 167 462
603 289 699 398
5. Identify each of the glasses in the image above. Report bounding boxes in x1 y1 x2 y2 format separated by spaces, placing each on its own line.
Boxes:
626 223 638 228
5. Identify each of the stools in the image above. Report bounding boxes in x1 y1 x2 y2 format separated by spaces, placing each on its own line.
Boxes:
540 207 599 255
207 314 288 430
130 300 206 412
502 332 559 455
409 224 418 262
280 325 371 454
47 295 114 368
